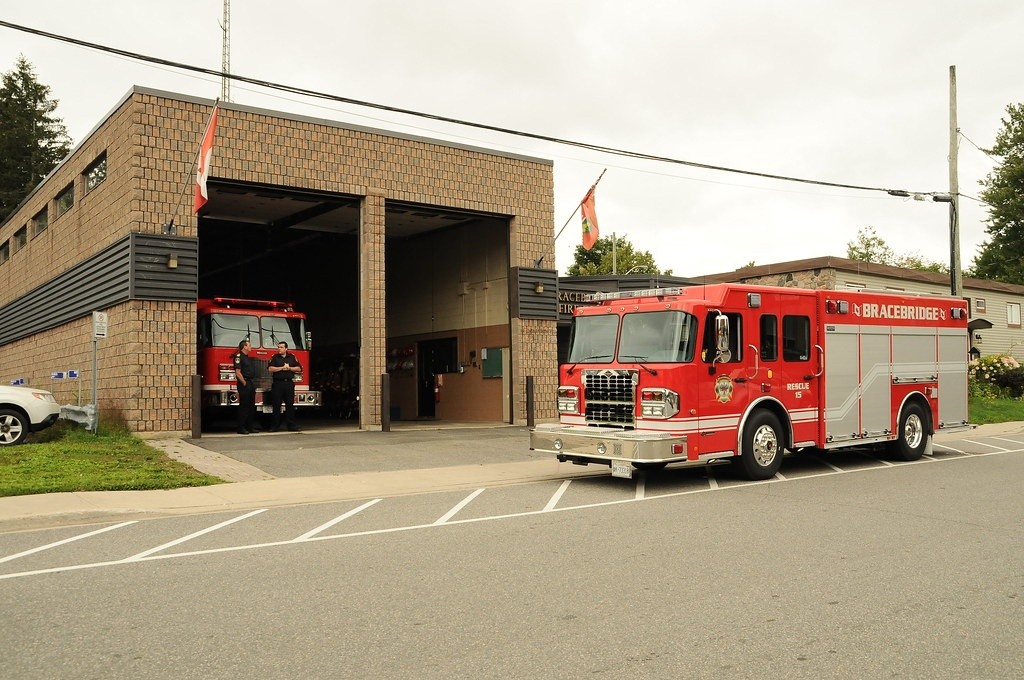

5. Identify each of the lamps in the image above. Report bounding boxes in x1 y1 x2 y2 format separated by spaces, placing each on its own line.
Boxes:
167 253 177 269
975 335 982 344
535 280 544 294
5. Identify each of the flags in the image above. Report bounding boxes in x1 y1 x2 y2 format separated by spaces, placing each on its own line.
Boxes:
581 185 599 250
193 106 218 215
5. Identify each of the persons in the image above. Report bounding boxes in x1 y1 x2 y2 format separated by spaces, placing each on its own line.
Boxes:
267 342 302 432
234 340 260 434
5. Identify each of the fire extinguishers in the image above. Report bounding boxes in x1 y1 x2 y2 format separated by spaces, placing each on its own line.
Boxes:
435 384 440 402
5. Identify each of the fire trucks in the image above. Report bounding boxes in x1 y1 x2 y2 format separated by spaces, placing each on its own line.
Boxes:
554 283 969 481
197 296 321 430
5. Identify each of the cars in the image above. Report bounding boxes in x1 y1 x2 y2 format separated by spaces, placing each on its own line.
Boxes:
0 385 62 447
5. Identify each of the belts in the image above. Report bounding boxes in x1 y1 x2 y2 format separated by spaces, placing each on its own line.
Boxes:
273 378 292 382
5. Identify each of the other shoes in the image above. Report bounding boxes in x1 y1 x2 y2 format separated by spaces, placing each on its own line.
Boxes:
268 426 280 431
287 425 302 431
237 429 259 434
